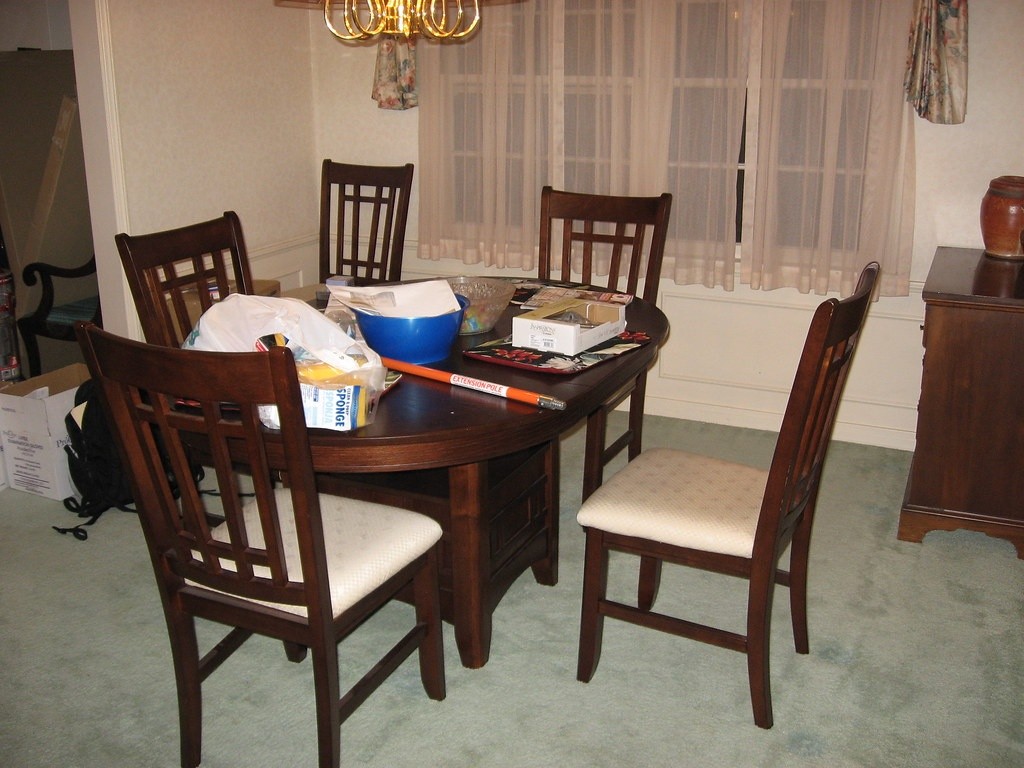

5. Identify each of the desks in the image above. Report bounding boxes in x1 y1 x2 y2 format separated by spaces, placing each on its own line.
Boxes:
175 277 671 669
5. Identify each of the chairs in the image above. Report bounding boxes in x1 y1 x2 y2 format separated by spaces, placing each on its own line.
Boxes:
115 211 255 349
576 261 880 730
73 319 446 768
538 186 672 504
16 254 103 377
319 159 414 286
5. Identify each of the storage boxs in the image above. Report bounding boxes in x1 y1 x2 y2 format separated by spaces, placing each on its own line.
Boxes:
166 280 280 348
0 364 92 502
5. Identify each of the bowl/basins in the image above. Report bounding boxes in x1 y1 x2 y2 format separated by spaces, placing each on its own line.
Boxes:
442 276 516 335
352 294 470 366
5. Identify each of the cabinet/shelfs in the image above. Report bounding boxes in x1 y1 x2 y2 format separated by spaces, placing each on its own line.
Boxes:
896 246 1024 559
